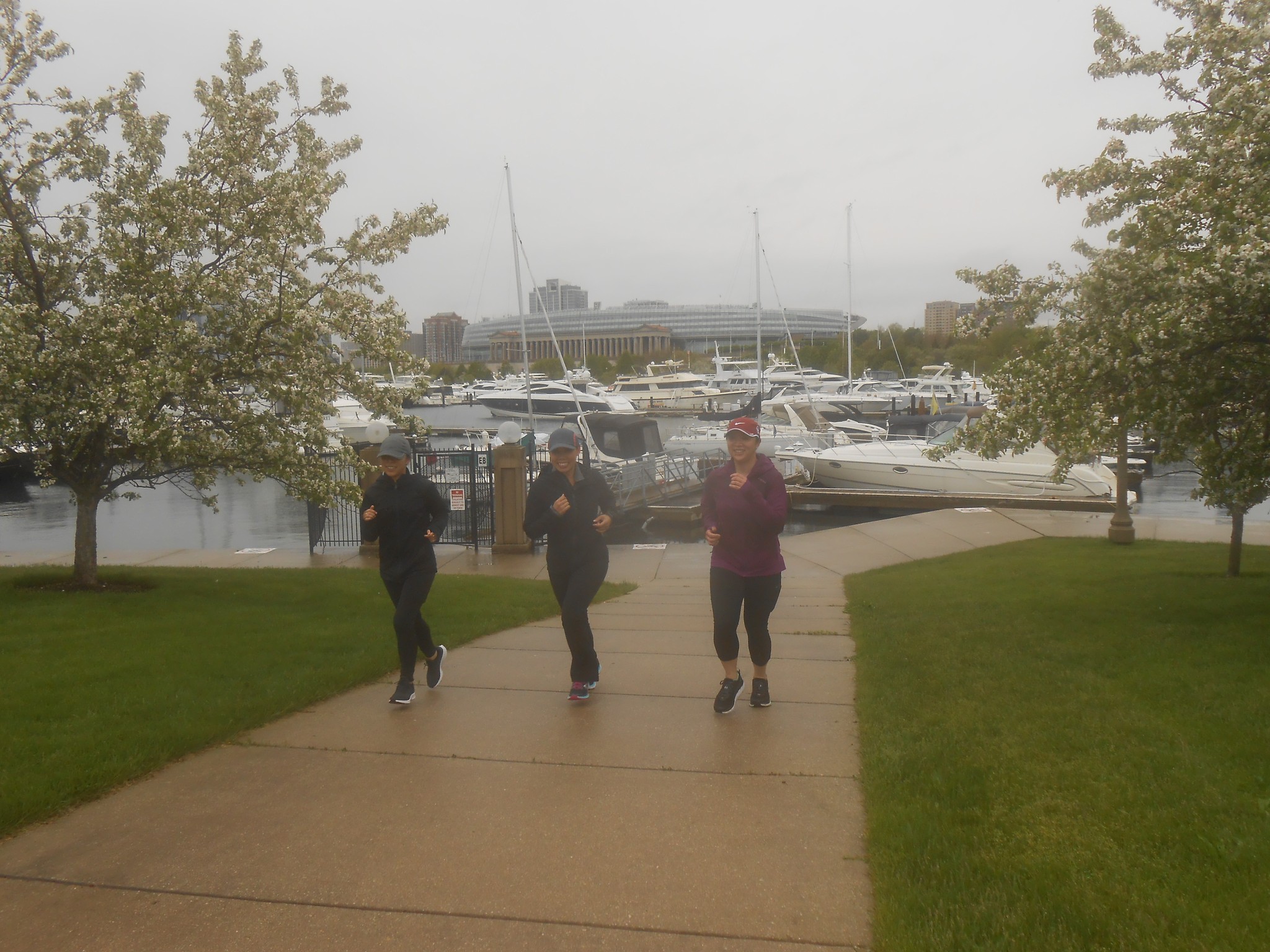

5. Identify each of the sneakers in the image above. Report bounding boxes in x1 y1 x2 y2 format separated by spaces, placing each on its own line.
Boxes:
424 645 447 688
389 679 416 704
750 674 771 707
589 663 601 689
714 669 744 713
568 683 590 700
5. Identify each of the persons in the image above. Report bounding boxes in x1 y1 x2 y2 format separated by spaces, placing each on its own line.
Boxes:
523 428 617 700
702 418 788 714
1140 430 1160 479
360 435 447 704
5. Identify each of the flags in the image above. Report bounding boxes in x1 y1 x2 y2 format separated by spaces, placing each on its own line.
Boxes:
930 390 939 415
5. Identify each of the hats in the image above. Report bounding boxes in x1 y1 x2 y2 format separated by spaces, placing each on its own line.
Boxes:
376 435 411 459
724 418 760 438
548 428 580 453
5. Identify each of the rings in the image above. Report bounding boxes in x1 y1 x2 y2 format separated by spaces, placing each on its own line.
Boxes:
433 535 435 537
365 513 367 515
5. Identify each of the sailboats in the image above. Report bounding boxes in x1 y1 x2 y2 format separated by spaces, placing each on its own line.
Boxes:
415 162 702 521
662 208 836 459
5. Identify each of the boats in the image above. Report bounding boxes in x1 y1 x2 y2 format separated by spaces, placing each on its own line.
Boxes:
771 404 1155 508
164 340 850 454
753 362 993 412
